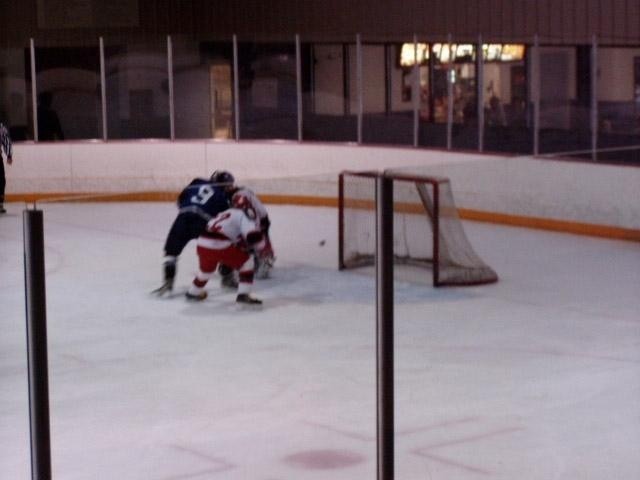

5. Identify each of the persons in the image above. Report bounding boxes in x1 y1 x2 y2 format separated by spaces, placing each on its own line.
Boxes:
0 123 13 213
227 184 274 277
152 171 238 296
186 198 268 306
463 96 530 129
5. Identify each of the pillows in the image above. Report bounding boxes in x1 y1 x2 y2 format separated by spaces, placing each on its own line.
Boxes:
216 173 234 191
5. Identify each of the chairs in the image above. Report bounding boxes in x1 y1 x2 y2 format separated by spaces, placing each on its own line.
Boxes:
236 294 262 305
151 280 173 297
254 254 274 278
185 290 207 301
222 277 238 288
